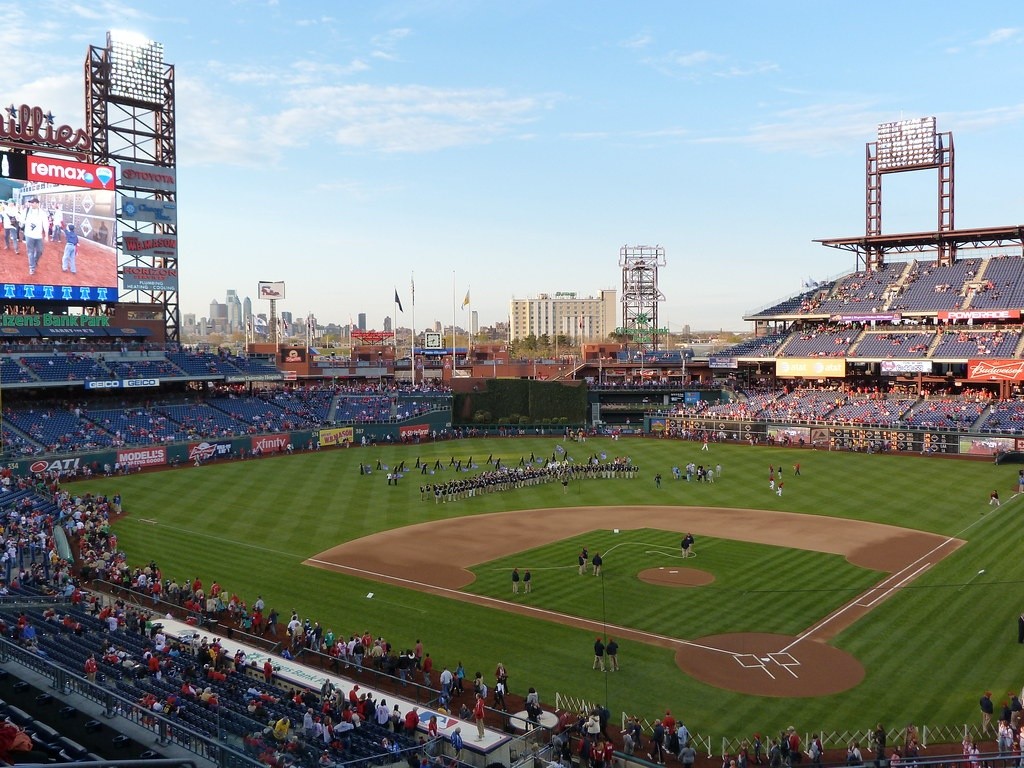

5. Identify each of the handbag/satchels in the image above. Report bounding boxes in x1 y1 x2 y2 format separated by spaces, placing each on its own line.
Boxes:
474 680 480 693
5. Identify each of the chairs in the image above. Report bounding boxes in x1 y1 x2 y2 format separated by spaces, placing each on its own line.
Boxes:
0 341 454 768
586 255 1024 436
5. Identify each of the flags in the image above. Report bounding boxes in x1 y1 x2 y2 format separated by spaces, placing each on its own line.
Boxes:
283 318 288 332
410 274 415 304
307 317 310 341
246 317 251 330
461 291 471 309
277 318 281 338
255 327 266 335
253 317 266 326
395 290 403 313
311 320 315 339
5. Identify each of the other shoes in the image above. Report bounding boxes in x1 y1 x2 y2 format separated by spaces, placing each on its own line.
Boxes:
647 753 652 759
474 738 483 742
657 762 665 764
50 239 54 242
30 268 35 274
615 667 619 671
58 240 62 242
601 670 606 672
4 246 9 249
16 250 20 254
593 667 599 669
610 669 613 671
477 734 485 738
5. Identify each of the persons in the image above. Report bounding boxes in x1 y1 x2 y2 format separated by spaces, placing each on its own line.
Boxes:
522 569 532 594
582 547 588 572
0 197 80 274
592 552 602 576
687 533 694 555
607 638 619 672
512 568 519 593
1 466 1024 767
593 639 607 672
681 536 688 558
579 554 584 575
0 259 1024 505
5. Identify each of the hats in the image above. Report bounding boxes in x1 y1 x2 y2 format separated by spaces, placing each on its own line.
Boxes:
609 637 613 640
6 199 15 203
28 198 40 203
666 709 671 715
753 732 760 738
596 637 602 641
269 720 274 727
354 685 361 689
787 726 795 731
